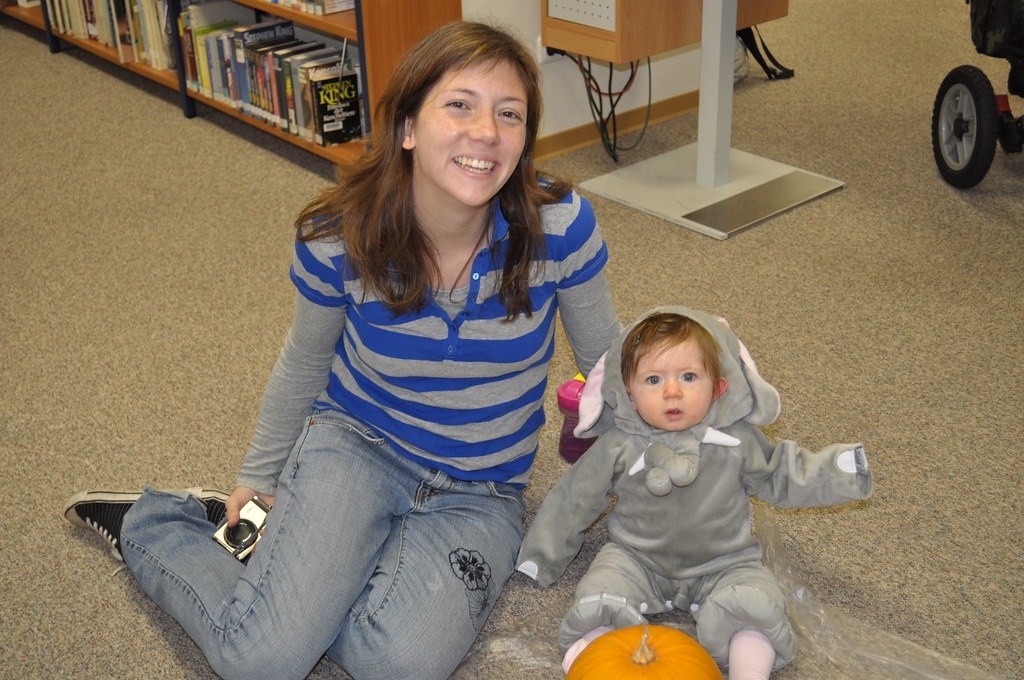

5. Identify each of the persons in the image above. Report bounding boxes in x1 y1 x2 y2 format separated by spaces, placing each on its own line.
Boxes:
64 22 624 680
515 304 873 680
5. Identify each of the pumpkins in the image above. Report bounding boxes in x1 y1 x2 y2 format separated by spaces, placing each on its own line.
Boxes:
566 624 725 680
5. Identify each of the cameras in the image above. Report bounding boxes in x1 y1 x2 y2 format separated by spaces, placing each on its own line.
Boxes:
212 495 272 560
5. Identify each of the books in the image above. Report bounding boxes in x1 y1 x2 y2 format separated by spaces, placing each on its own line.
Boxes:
17 0 364 149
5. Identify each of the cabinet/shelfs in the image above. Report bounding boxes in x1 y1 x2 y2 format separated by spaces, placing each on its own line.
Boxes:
0 0 789 189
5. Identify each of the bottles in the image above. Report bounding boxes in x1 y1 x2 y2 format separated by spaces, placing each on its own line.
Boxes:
556 373 597 465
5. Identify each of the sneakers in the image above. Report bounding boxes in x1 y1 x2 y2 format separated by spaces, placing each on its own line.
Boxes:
62 489 234 576
